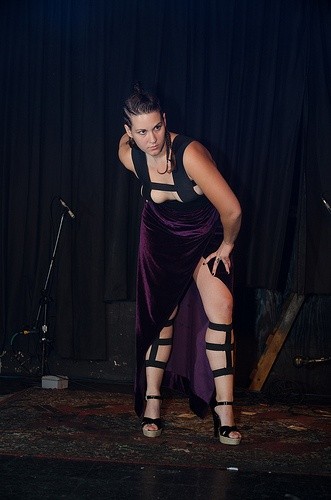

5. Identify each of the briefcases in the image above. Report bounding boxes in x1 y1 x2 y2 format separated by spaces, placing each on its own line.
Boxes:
42 374 68 390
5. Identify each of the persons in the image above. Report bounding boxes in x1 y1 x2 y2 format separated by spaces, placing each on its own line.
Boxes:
118 81 244 446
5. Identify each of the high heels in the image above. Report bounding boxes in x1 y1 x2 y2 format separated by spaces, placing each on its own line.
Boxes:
210 398 242 446
141 395 162 437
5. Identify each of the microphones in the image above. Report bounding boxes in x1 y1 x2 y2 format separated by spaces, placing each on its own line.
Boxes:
321 197 331 215
58 198 76 220
